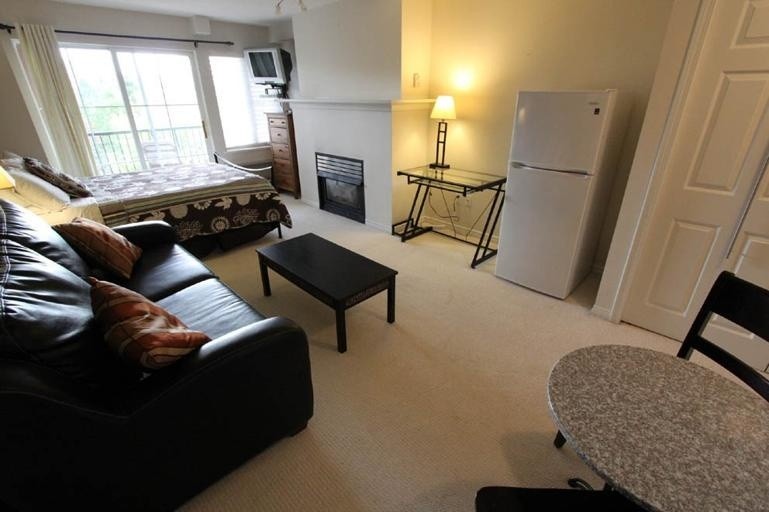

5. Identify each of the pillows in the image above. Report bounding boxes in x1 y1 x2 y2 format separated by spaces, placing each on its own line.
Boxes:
84 274 213 375
8 165 72 212
22 155 94 198
50 215 143 280
0 148 25 168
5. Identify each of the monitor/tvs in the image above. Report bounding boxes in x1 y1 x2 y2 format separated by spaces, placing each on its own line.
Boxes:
243 47 293 86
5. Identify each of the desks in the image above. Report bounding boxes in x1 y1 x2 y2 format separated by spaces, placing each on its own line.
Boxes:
544 340 769 512
390 165 507 270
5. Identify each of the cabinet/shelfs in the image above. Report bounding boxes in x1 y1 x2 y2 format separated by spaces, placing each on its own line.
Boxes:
265 112 301 200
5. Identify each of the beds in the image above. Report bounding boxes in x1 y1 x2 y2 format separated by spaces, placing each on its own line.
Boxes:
0 160 294 258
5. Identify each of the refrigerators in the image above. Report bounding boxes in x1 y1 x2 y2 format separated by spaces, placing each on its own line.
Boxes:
492 87 623 300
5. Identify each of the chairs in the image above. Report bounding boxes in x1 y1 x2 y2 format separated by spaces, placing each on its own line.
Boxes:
674 269 768 407
471 485 662 512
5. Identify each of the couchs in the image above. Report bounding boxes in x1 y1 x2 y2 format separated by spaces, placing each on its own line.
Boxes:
0 192 316 512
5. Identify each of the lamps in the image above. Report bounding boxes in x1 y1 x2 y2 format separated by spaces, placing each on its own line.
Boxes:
273 0 309 17
428 95 459 171
